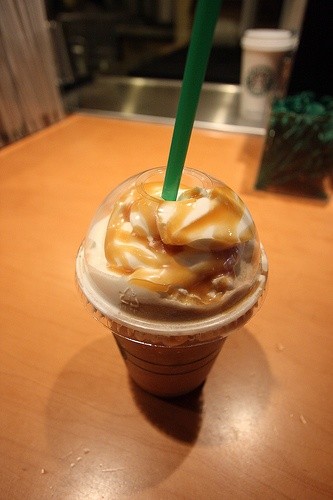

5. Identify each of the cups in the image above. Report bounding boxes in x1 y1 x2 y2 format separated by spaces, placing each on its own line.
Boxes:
240 30 296 120
74 165 268 397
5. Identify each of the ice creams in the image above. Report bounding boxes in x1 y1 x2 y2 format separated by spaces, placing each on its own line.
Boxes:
75 181 269 397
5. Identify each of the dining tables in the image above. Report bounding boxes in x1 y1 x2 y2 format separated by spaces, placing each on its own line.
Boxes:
1 106 331 500
